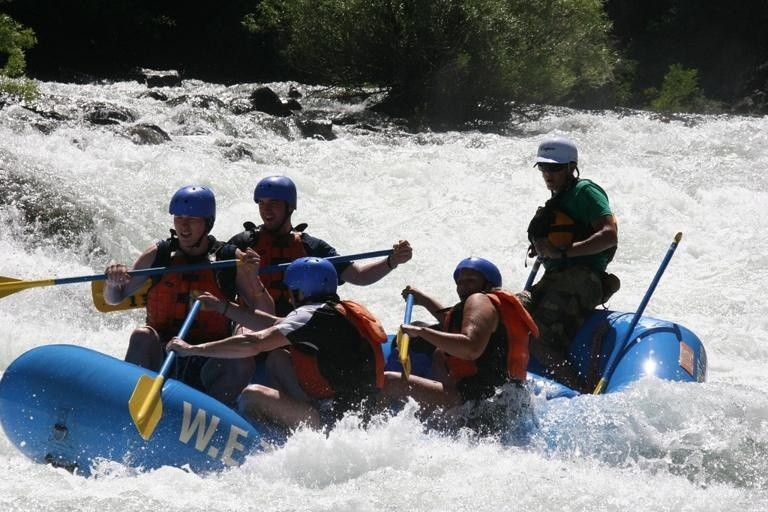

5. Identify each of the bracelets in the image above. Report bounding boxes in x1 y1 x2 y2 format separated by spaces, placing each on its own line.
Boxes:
561 248 569 260
252 285 266 297
386 255 397 271
220 301 230 315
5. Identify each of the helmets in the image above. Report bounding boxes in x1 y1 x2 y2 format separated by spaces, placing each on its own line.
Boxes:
453 256 503 289
253 175 297 210
535 136 578 168
283 254 339 299
168 184 216 221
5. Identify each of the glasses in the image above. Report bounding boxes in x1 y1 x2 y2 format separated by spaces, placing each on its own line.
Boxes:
538 163 563 173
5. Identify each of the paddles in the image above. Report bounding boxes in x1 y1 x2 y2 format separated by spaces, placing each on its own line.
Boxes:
394 285 414 381
91 248 399 312
128 289 206 441
1 258 244 299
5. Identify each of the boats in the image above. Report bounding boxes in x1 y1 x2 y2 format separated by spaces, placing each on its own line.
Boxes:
0 303 706 482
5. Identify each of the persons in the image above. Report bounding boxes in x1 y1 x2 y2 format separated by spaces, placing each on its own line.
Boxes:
164 257 387 446
102 184 276 409
226 174 413 383
514 137 620 395
378 256 540 432
